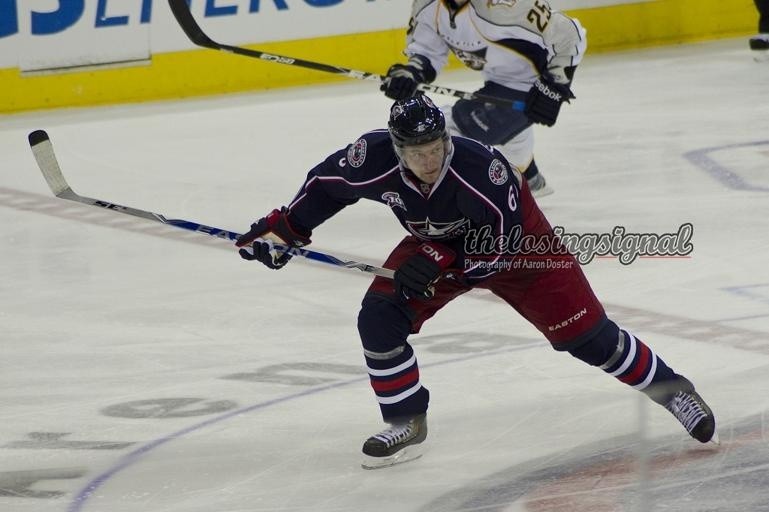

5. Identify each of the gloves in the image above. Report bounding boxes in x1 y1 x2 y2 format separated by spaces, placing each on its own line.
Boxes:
234 205 313 271
392 242 463 303
524 66 576 129
380 54 436 103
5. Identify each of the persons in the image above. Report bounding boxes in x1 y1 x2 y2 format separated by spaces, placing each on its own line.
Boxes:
234 92 721 461
750 0 768 63
382 0 587 199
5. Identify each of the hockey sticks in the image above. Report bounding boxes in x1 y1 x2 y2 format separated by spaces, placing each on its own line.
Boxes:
168 0 524 112
29 130 396 281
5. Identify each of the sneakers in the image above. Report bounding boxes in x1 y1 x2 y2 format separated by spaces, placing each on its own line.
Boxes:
362 409 427 457
526 173 545 194
664 374 714 444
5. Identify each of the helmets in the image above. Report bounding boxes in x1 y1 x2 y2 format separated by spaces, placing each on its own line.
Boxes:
387 94 453 169
